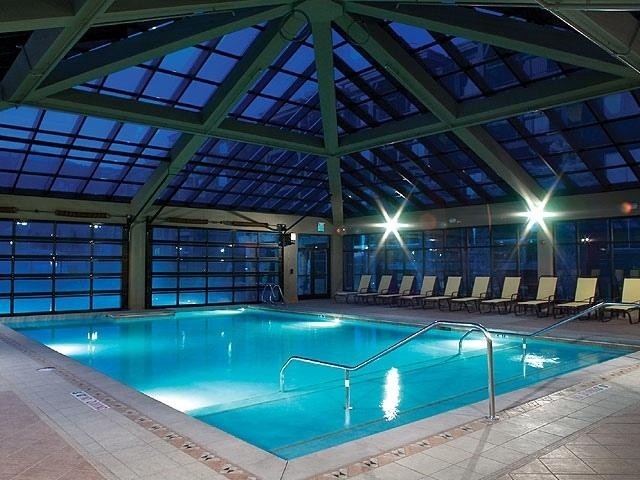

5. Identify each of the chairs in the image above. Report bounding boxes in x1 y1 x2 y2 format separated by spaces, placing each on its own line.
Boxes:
354 275 393 306
334 275 372 304
552 276 598 320
514 275 558 318
599 277 640 324
449 276 490 314
396 275 437 310
423 276 461 311
478 275 521 315
376 275 415 308
